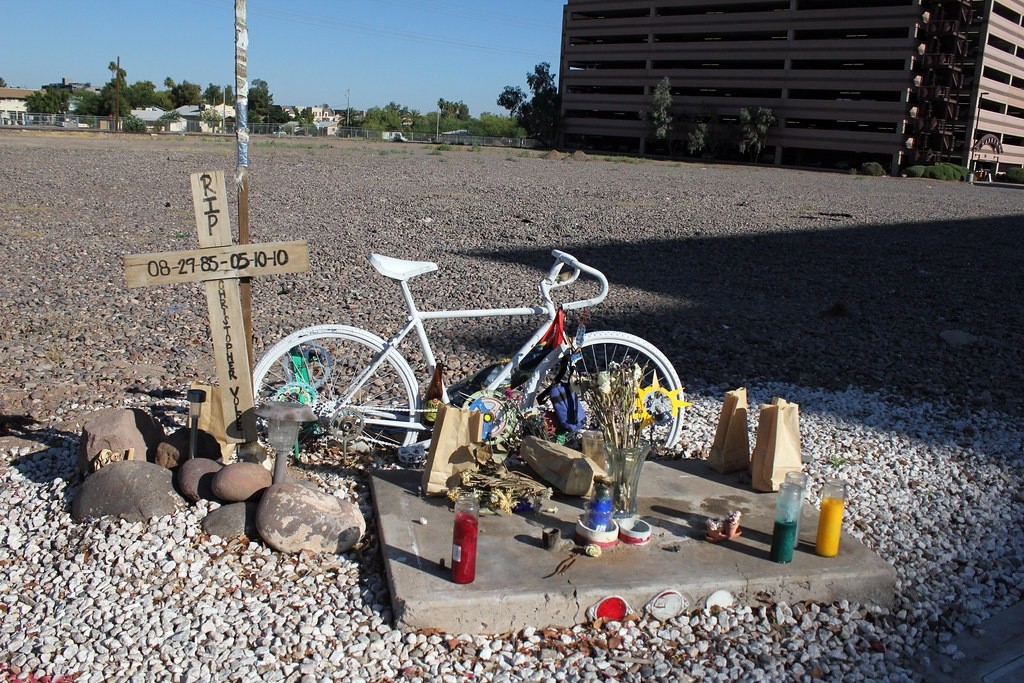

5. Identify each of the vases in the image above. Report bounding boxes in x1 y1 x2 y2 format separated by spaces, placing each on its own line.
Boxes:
602 436 652 519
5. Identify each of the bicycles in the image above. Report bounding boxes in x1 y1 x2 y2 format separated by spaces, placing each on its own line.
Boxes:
250 249 685 462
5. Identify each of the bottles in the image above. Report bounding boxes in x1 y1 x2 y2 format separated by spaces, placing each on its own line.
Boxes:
420 363 443 426
589 488 610 531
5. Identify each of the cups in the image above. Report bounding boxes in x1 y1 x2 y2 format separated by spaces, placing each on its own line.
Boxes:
709 522 739 535
451 497 480 584
785 472 808 547
543 528 561 551
582 431 605 496
815 478 849 557
771 483 802 564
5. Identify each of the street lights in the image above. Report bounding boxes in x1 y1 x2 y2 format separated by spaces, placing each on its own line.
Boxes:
968 92 990 183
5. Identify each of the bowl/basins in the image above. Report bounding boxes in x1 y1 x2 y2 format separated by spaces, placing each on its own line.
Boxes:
617 518 651 545
575 513 618 548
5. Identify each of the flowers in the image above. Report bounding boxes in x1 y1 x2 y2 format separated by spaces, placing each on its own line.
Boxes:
569 357 645 449
500 388 557 465
446 459 553 517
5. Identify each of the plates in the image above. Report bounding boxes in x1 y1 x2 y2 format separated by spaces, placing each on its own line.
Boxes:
589 595 634 623
705 527 742 542
645 589 688 621
697 589 742 617
